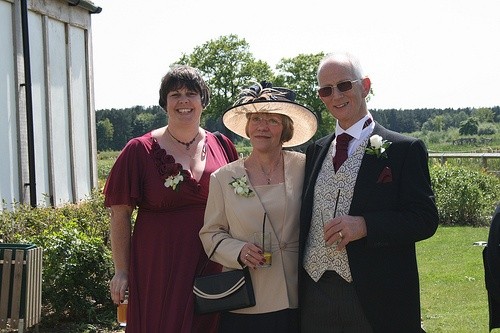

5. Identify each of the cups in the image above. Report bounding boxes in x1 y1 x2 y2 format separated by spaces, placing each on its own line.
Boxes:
117 291 129 327
321 210 343 247
254 232 272 267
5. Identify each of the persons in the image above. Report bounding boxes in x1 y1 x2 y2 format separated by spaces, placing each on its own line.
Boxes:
483 206 500 331
295 55 438 333
199 81 318 333
103 66 237 333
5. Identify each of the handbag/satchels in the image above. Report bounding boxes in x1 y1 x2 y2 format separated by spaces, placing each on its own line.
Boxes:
192 237 256 315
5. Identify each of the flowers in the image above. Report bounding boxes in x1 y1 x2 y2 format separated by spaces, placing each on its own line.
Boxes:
364 134 392 158
163 171 185 192
228 176 254 198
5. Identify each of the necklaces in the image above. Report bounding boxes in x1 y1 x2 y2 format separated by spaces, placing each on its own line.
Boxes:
264 159 282 185
167 128 199 150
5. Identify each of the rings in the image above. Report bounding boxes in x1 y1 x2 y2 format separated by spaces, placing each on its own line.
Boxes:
339 232 343 238
245 254 248 260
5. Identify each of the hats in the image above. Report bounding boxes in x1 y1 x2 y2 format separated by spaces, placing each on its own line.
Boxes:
222 80 318 147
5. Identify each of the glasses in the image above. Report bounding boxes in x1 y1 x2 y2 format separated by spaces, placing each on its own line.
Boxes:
317 78 364 98
248 114 284 128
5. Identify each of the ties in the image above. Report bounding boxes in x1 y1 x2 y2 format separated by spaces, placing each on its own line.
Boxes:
333 118 373 175
